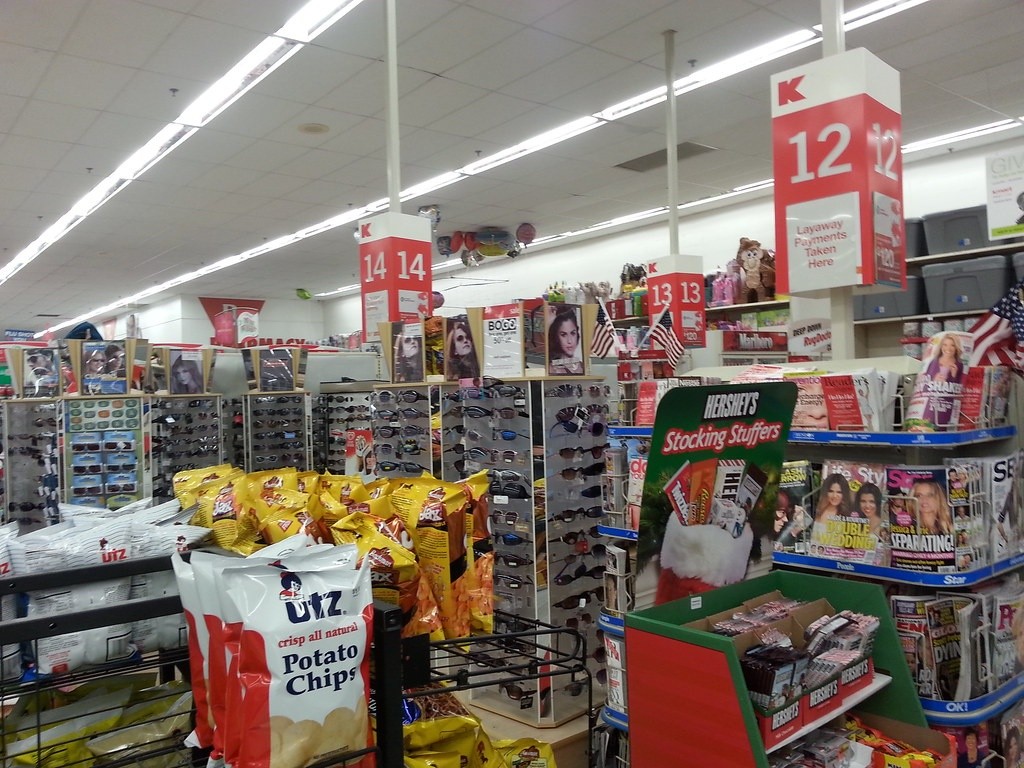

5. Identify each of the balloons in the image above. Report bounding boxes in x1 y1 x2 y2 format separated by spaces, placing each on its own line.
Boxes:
415 206 536 309
297 289 312 301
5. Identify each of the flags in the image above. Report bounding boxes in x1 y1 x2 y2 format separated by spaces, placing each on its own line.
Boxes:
648 310 684 370
590 304 615 359
969 274 1024 380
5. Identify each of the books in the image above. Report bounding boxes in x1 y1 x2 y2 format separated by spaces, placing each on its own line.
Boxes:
603 333 1024 768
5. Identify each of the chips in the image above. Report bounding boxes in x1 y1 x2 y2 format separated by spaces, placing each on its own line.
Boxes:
396 554 492 638
268 694 369 768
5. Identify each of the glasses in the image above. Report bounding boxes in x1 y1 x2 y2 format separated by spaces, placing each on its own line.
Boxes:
584 442 611 459
150 390 433 484
27 355 43 363
549 404 611 437
92 358 104 363
445 425 488 442
443 406 493 419
401 337 418 345
484 385 526 399
492 428 532 442
104 461 138 473
545 384 583 399
446 443 541 700
71 483 103 496
153 485 173 498
548 446 585 460
7 418 57 459
589 386 610 399
69 463 102 475
71 441 102 452
490 407 529 420
108 356 124 361
443 388 485 401
105 481 137 493
8 500 45 524
102 440 135 452
547 462 614 696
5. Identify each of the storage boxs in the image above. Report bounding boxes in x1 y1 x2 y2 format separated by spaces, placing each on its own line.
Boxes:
849 200 1024 325
747 656 874 768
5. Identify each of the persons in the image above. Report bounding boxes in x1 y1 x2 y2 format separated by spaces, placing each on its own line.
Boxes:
393 329 422 384
81 341 126 380
28 347 59 386
809 467 974 573
446 320 479 380
1003 724 1024 768
917 333 964 431
170 353 203 394
957 726 986 768
546 303 584 375
788 379 829 432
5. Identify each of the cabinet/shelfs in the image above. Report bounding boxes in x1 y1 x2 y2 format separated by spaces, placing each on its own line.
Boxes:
852 241 1024 327
596 423 1024 729
622 569 932 768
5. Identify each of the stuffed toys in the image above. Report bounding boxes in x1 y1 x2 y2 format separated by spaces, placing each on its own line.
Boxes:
735 237 776 302
